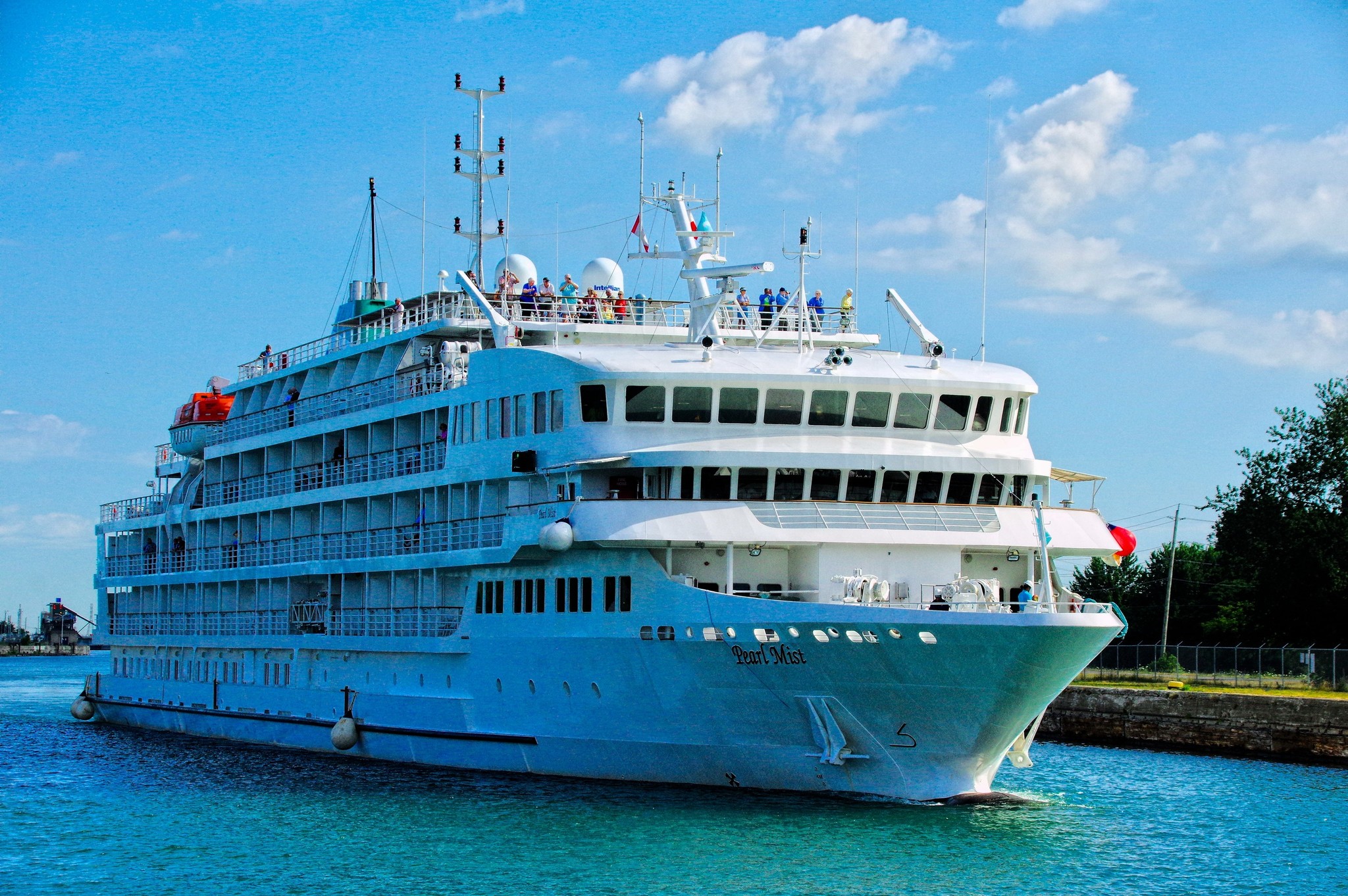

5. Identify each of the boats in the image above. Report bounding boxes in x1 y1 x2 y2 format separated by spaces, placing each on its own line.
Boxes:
168 392 235 455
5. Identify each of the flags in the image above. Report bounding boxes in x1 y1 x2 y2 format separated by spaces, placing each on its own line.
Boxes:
631 212 649 252
688 210 713 240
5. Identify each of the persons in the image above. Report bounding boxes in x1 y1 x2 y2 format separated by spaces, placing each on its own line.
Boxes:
259 345 273 369
222 530 238 546
463 273 478 319
389 298 404 333
404 502 425 551
497 267 627 324
840 288 853 333
172 536 185 571
808 290 825 332
142 538 156 574
1018 581 1037 613
436 423 447 440
929 589 949 611
793 291 810 331
332 439 344 473
737 287 749 329
759 287 790 331
284 387 300 428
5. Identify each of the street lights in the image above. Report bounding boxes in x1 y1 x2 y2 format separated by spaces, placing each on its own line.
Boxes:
17 603 23 640
55 613 71 645
4 610 9 639
38 616 40 634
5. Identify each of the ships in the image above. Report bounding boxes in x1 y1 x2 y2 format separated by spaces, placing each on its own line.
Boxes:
83 71 1133 806
